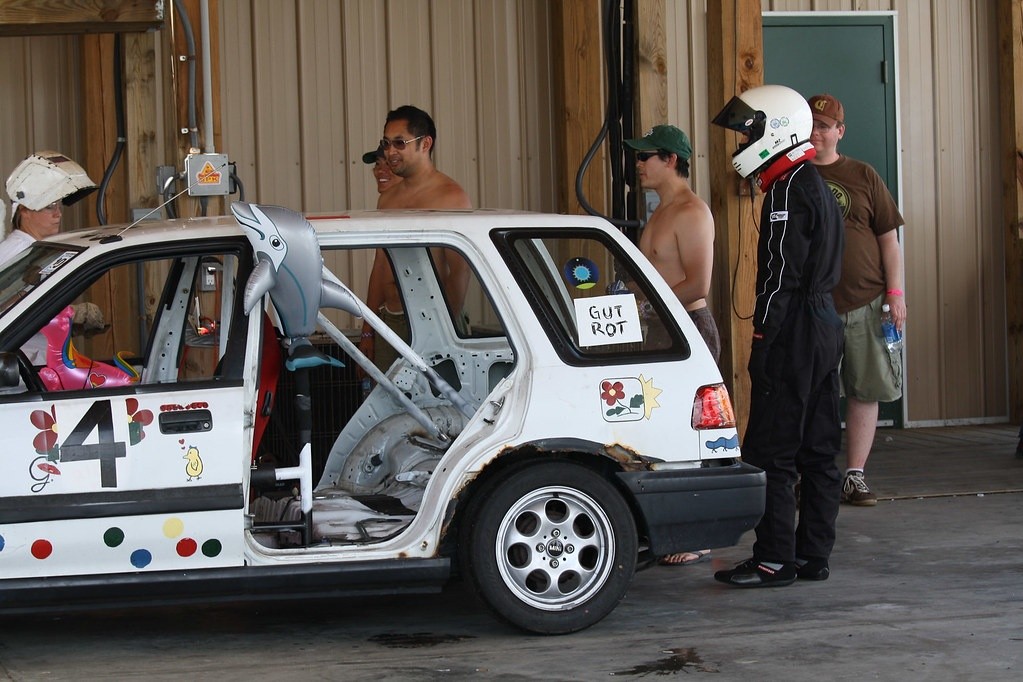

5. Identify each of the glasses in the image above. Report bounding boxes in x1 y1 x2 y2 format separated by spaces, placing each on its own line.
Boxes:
637 152 672 161
380 135 431 150
812 122 840 133
40 201 64 214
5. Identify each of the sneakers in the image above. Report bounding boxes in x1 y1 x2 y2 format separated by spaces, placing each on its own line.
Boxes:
840 470 878 506
796 554 830 580
714 558 798 589
793 473 801 511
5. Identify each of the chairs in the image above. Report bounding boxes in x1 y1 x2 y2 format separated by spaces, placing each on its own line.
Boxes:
213 312 283 459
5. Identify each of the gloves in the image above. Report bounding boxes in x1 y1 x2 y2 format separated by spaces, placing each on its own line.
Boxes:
747 350 774 395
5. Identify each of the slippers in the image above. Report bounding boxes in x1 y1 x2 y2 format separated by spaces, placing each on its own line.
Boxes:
661 550 712 566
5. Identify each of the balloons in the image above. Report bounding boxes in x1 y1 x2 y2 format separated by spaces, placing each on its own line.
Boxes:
228 202 364 370
36 307 140 391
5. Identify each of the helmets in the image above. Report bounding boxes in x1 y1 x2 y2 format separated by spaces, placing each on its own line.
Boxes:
5 149 99 223
732 84 816 194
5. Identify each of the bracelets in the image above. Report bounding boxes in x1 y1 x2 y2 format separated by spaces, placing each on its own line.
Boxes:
887 288 905 298
361 330 373 338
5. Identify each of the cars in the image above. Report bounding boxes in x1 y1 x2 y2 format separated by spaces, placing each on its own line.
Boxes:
0 209 768 636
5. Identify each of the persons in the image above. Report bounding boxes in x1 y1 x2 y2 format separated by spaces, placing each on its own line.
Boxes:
362 146 404 192
0 152 110 365
606 124 722 565
355 104 474 396
793 95 908 509
709 84 846 588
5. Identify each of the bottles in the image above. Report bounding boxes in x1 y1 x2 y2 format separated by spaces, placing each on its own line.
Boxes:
880 304 903 355
361 373 372 402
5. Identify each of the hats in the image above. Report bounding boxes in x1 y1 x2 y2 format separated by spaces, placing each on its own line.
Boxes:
808 94 845 124
623 124 693 161
362 145 386 164
